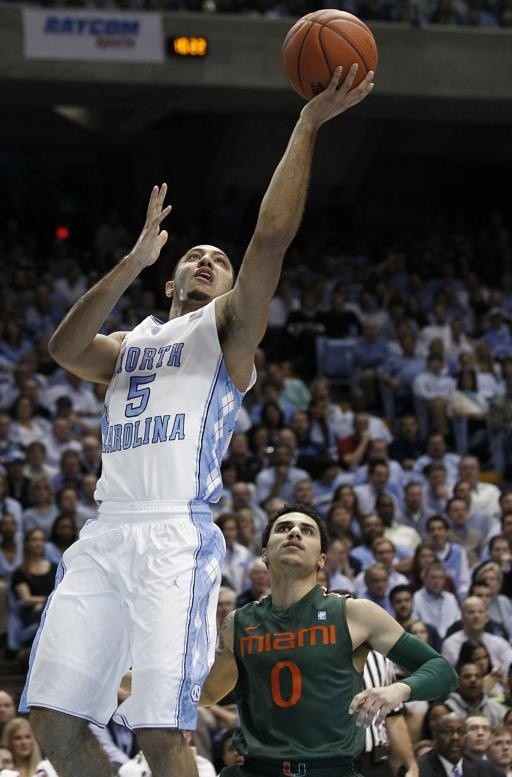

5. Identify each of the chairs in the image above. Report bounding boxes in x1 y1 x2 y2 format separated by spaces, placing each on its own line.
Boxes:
313 332 512 478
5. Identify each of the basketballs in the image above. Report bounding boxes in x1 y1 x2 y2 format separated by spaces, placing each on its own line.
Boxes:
281 8 380 100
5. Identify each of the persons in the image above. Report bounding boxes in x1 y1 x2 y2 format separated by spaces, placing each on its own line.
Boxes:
17 2 511 28
0 61 512 777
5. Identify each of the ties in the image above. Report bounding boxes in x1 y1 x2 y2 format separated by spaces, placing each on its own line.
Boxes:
452 765 461 777
